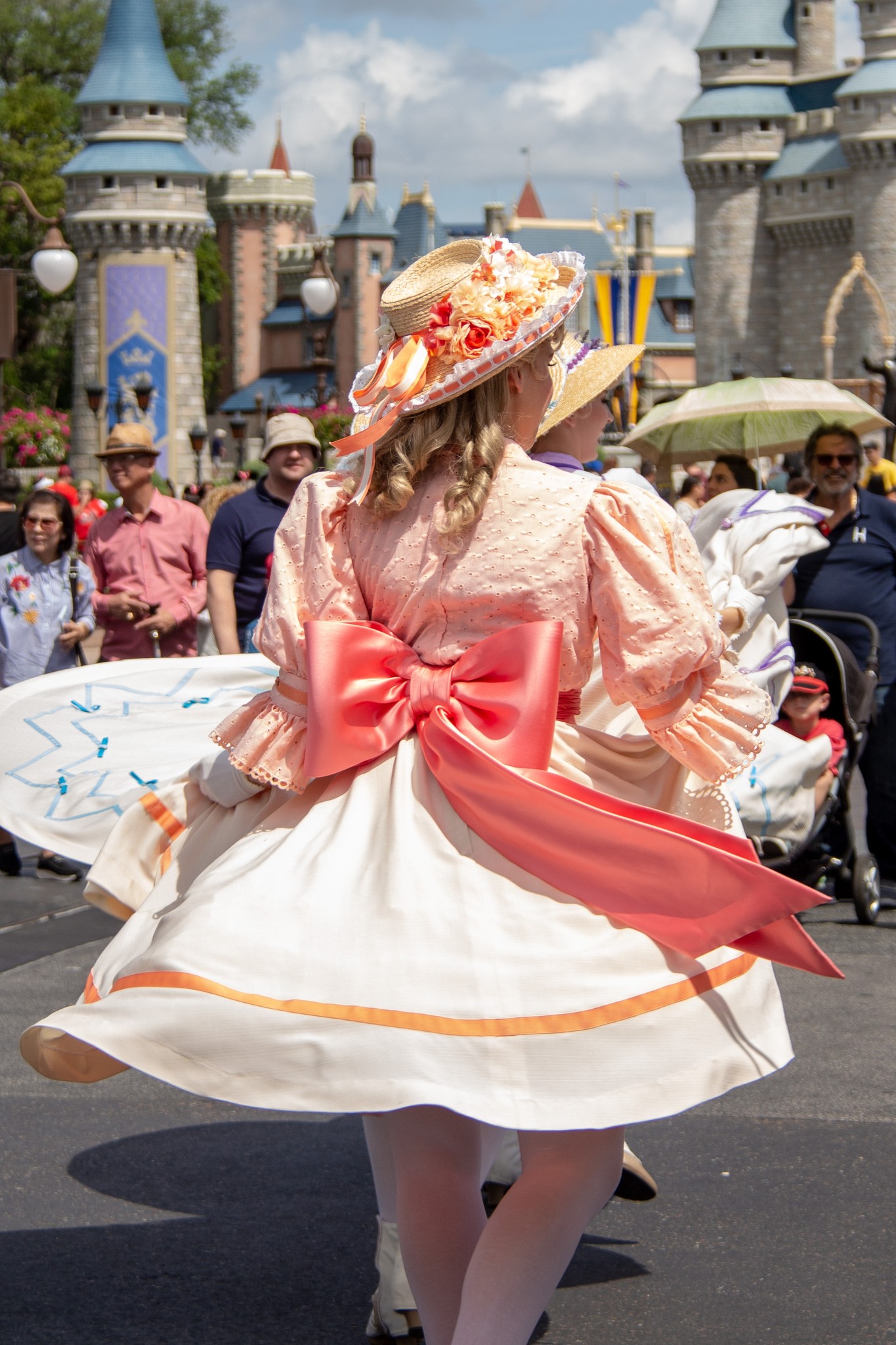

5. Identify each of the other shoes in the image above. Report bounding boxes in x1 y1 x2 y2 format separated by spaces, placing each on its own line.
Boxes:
615 1149 657 1202
834 877 855 902
408 1326 424 1340
485 1181 507 1205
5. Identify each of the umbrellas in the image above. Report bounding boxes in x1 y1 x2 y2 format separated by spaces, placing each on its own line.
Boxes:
617 376 896 491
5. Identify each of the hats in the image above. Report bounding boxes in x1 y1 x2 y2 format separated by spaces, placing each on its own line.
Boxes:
327 233 589 458
534 331 646 438
93 422 162 458
789 661 829 695
258 412 323 462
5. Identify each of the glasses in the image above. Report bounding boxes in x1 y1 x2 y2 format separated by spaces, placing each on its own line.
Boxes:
102 453 150 469
817 453 857 466
22 516 64 532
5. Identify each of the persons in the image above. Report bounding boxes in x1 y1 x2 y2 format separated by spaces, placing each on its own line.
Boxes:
29 465 110 554
786 422 896 906
209 411 323 655
747 663 845 857
21 238 847 1344
84 421 213 663
637 441 896 605
1 492 99 880
0 323 833 1336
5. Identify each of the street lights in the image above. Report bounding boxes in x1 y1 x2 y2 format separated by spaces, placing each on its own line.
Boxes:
0 181 80 364
300 241 341 425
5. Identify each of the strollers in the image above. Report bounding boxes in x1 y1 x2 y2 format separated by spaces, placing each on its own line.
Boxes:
759 606 879 914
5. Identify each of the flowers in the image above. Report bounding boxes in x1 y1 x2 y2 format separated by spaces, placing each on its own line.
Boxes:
420 234 559 365
